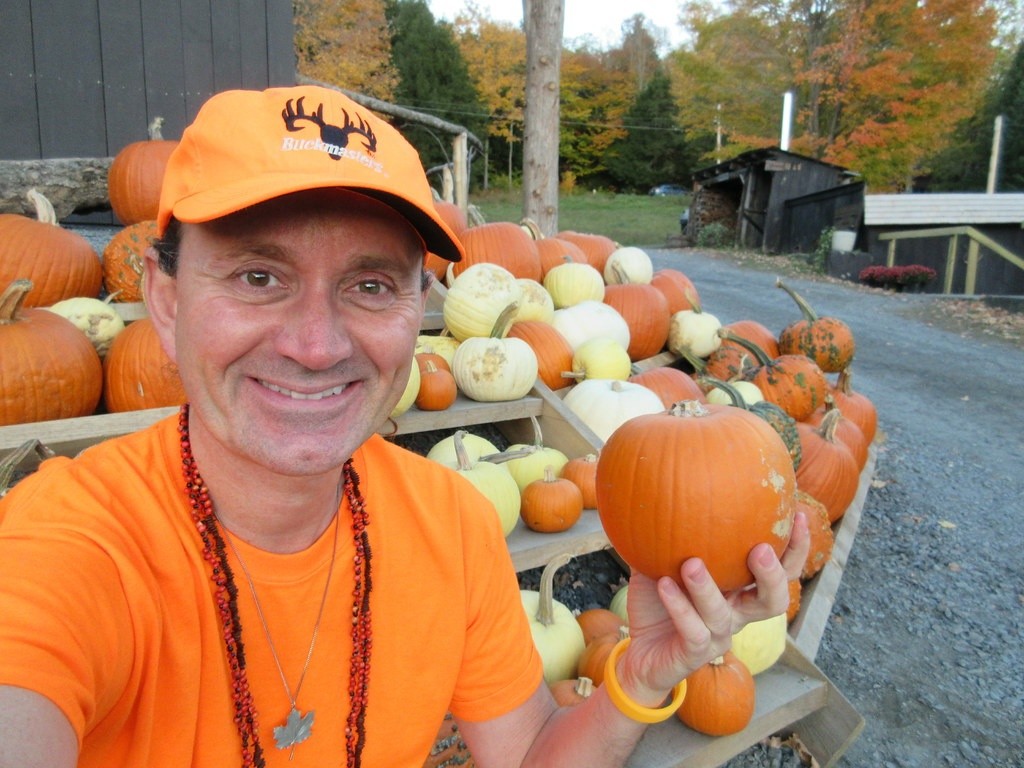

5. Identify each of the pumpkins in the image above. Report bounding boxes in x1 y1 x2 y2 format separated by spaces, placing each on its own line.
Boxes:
0 120 872 737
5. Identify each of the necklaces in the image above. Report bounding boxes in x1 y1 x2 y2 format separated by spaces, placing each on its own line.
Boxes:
176 402 374 768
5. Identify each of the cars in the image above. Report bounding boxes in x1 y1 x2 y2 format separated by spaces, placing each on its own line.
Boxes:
650 183 692 197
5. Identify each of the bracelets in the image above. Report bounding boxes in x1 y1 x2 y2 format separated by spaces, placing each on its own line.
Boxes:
603 638 687 724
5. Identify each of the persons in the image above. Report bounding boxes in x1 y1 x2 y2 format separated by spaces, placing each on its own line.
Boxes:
0 83 811 768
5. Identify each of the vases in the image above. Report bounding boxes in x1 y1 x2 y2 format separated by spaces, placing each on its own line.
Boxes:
868 283 923 294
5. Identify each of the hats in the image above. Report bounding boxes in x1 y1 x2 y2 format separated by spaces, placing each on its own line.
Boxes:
157 85 467 263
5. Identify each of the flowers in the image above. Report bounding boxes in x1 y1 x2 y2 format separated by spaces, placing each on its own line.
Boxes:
859 266 936 283
